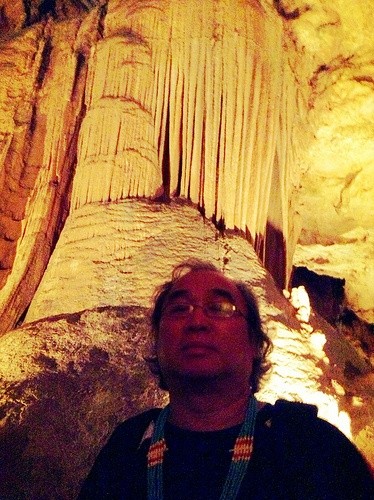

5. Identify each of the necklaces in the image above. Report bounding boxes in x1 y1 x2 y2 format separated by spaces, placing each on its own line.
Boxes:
147 392 259 500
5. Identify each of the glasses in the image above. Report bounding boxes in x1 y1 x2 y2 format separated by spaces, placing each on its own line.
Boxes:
161 301 249 322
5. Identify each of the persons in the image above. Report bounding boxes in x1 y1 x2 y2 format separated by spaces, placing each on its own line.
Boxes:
75 255 374 499
